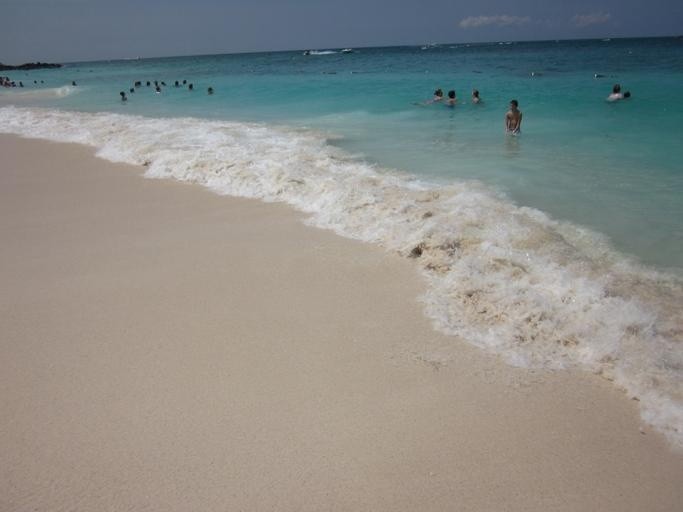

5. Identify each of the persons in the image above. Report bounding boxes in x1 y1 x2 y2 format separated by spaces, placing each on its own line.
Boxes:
410 89 444 108
504 100 523 136
471 89 481 104
0 75 79 89
445 90 459 107
118 79 214 101
606 84 623 102
623 91 631 100
